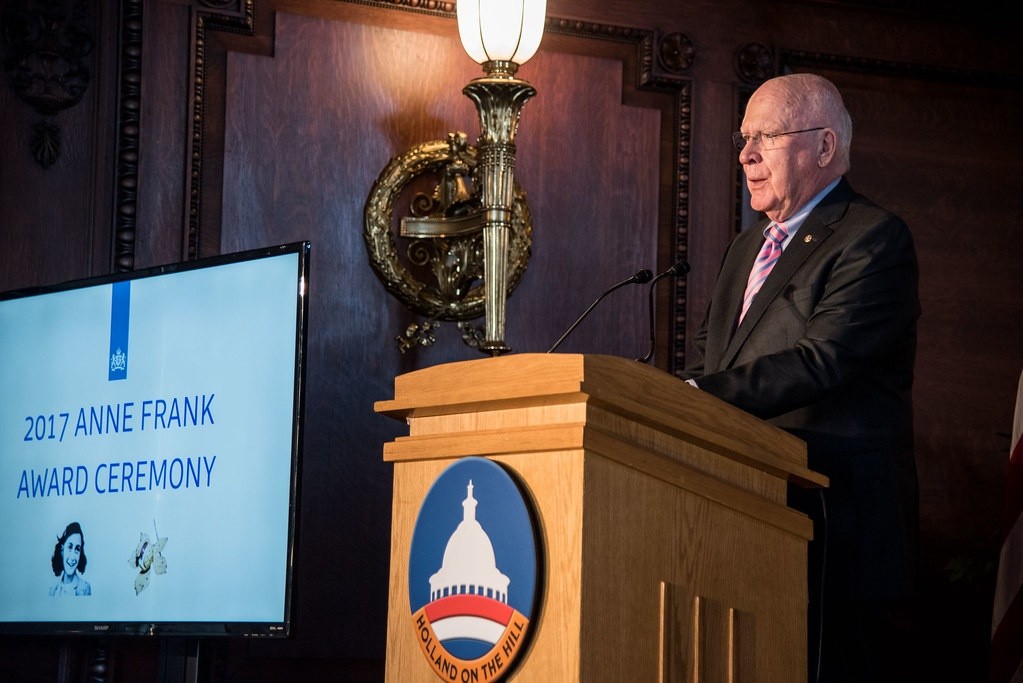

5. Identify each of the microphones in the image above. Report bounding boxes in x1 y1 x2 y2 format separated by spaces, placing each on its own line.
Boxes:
546 260 692 364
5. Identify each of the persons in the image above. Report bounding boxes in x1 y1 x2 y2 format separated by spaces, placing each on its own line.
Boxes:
677 73 922 683
48 522 91 597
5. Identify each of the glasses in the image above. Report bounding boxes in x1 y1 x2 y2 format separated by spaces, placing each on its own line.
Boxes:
732 127 826 152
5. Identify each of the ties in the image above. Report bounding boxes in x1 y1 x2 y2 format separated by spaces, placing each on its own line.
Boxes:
737 223 790 328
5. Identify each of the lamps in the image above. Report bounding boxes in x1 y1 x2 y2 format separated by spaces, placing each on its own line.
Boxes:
362 0 548 357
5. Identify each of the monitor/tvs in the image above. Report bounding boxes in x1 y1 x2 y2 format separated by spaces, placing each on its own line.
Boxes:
0 241 312 644
